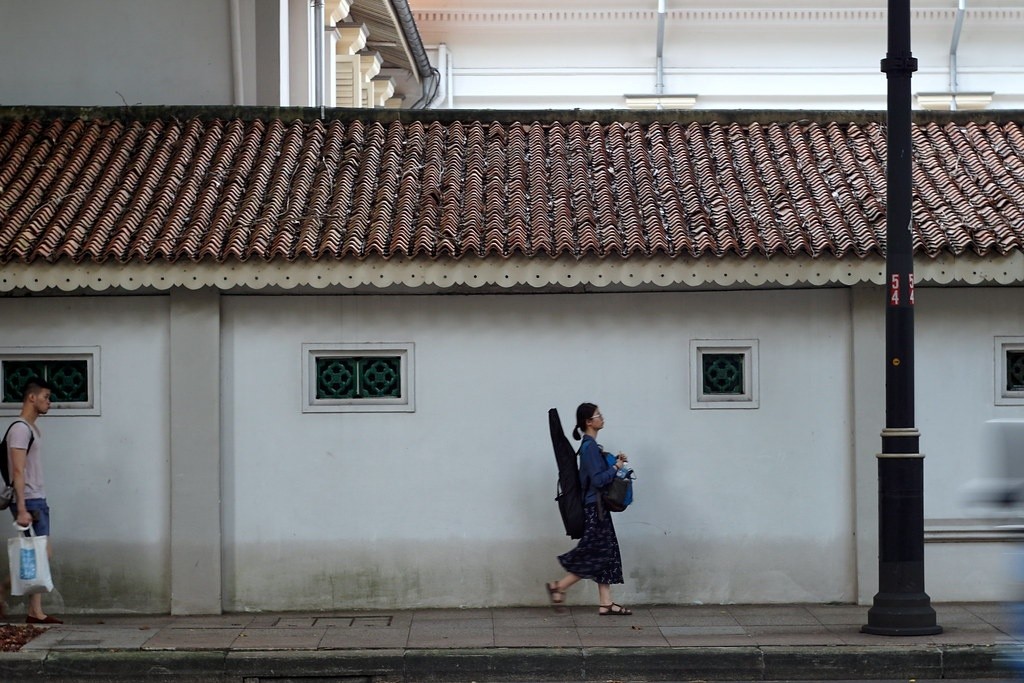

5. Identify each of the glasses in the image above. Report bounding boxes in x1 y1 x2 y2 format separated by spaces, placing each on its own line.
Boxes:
589 414 603 419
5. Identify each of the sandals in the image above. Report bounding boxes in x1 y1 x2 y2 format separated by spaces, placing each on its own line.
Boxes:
546 581 567 613
598 602 633 615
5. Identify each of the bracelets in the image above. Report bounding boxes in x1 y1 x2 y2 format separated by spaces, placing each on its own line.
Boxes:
613 463 619 469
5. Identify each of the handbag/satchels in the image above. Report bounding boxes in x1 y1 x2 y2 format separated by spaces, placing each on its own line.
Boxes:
7 521 54 596
0 420 34 510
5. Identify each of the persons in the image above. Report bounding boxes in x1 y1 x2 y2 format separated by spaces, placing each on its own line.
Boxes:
546 403 633 615
0 378 64 624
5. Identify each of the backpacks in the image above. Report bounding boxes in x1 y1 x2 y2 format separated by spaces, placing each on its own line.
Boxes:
583 439 637 512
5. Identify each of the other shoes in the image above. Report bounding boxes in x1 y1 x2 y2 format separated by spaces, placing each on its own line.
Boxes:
0 603 10 621
25 615 63 624
